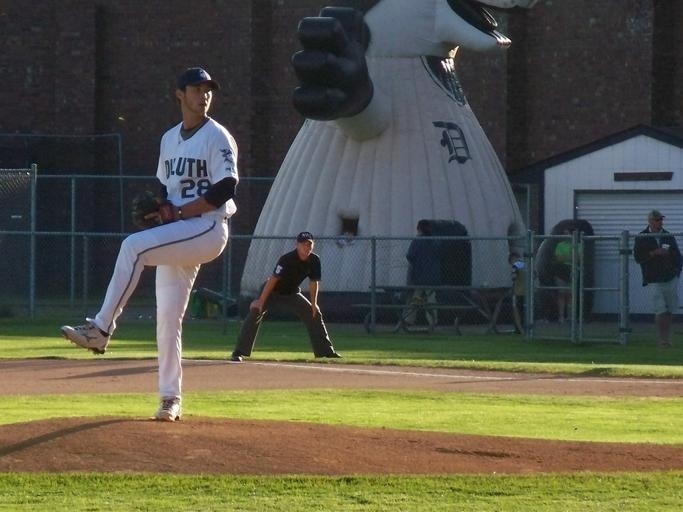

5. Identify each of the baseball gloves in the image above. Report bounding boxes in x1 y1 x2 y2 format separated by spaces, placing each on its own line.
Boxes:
130 190 165 228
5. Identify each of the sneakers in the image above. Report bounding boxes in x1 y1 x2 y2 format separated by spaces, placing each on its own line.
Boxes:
60 321 110 355
157 399 181 422
315 352 342 358
232 352 242 361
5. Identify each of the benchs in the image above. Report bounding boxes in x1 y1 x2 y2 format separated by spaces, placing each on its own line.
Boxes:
354 306 479 336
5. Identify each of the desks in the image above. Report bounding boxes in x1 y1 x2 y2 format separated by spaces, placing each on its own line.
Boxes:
369 284 512 336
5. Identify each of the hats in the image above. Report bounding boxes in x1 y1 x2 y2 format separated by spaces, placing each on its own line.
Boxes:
648 210 665 221
174 67 220 91
297 232 314 243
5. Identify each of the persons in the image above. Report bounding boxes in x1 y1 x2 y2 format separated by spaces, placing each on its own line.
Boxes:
554 229 583 324
399 220 441 327
634 211 683 348
61 68 238 422
232 232 342 362
509 253 529 335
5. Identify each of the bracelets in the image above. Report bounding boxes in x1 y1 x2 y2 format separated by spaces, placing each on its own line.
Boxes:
178 207 184 220
